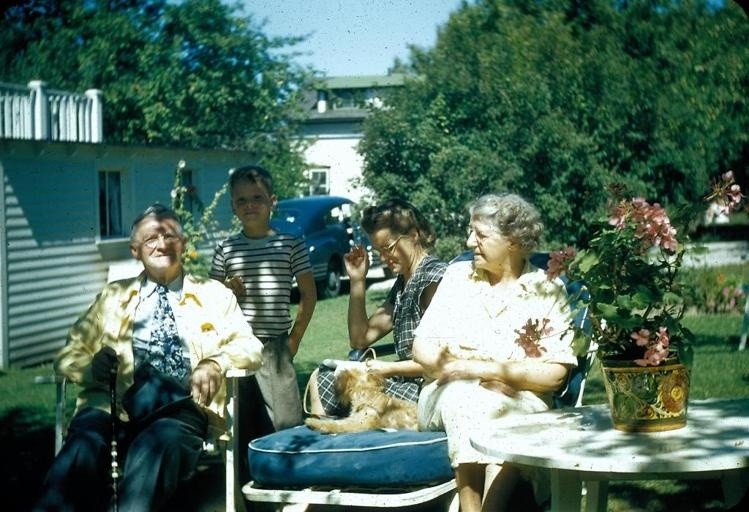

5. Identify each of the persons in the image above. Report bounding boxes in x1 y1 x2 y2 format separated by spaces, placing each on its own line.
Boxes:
410 192 582 512
205 165 318 434
20 202 265 511
309 199 449 420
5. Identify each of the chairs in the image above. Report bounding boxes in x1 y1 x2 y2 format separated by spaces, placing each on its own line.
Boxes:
243 251 600 511
51 331 241 512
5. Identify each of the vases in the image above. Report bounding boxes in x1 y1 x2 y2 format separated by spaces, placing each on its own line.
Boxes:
597 355 687 431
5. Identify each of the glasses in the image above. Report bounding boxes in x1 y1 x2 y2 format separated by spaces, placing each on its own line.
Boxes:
370 235 409 256
139 229 185 249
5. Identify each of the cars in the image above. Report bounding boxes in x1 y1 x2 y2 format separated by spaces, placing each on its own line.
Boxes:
267 195 398 298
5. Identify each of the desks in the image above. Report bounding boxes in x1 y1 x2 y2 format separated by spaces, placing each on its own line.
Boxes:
470 405 748 511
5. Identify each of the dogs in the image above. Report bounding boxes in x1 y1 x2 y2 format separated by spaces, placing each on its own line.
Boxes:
304 365 418 435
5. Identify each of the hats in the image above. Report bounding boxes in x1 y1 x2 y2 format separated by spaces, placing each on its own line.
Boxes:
121 379 193 432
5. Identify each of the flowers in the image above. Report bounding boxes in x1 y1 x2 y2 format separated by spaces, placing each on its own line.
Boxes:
542 164 745 368
